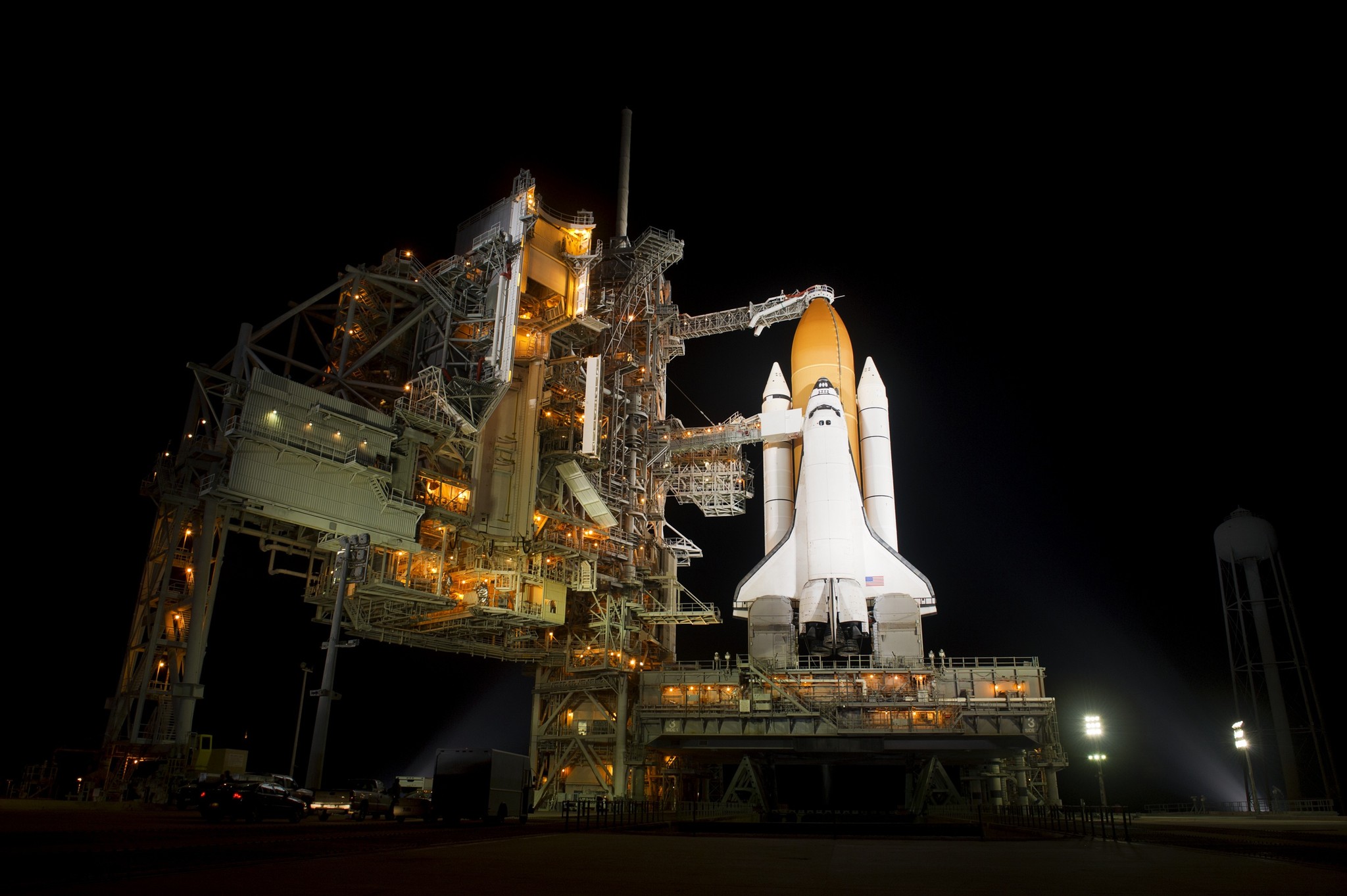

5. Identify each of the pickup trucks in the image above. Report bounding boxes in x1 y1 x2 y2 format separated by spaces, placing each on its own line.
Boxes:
314 779 395 821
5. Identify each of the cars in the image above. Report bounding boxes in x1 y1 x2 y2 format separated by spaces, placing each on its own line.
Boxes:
394 789 432 824
200 782 307 822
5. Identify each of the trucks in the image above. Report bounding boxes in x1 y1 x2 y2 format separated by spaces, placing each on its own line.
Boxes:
432 749 537 827
239 773 312 810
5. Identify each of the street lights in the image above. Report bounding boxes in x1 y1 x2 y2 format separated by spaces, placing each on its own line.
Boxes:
1085 713 1110 818
1232 721 1261 812
303 532 370 786
